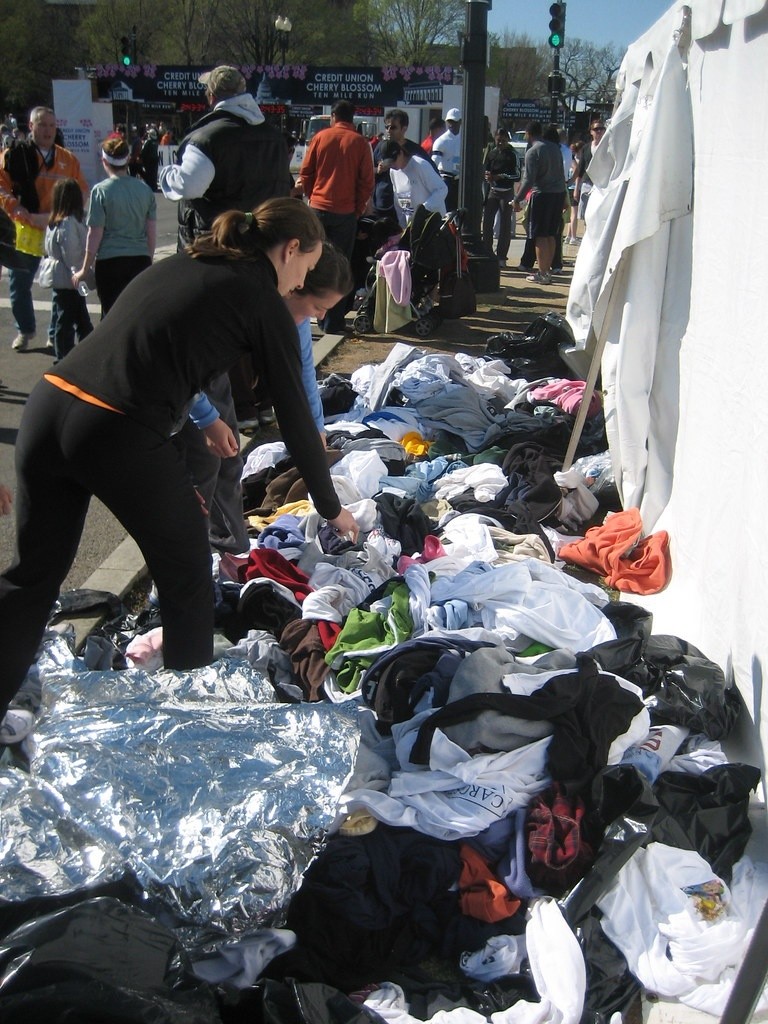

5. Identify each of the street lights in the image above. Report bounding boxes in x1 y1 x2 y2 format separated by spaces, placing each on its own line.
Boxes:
273 15 292 136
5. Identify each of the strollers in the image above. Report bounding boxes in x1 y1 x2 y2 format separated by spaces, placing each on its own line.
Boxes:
351 203 469 338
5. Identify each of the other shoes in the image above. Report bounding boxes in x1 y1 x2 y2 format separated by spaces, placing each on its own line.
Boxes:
236 417 258 430
259 408 275 423
318 325 353 335
564 236 582 246
498 260 506 268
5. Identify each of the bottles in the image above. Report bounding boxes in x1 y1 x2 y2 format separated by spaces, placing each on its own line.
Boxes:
70 265 89 296
508 200 527 208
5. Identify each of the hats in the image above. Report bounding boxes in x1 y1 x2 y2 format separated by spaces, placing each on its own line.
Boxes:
199 64 246 98
147 129 157 140
445 107 462 121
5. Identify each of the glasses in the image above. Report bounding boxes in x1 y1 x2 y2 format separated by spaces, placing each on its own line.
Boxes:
385 124 401 130
590 127 605 131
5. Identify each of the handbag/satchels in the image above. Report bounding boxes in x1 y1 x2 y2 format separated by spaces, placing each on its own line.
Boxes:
439 272 476 319
374 272 412 333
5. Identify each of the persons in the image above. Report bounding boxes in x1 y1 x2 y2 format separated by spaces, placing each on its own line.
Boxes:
159 65 291 255
0 105 91 352
44 177 95 365
0 196 359 744
54 127 65 149
113 119 179 193
0 113 33 153
72 138 157 322
292 99 609 335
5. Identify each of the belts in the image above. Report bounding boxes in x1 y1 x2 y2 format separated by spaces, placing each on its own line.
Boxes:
440 174 460 180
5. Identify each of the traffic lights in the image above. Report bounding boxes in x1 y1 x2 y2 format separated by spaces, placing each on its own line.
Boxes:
548 2 567 48
121 36 131 67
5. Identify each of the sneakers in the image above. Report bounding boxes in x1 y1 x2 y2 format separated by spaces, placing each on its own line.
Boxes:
0 710 35 743
553 268 562 275
11 330 36 351
518 264 532 271
47 340 55 352
526 271 552 285
